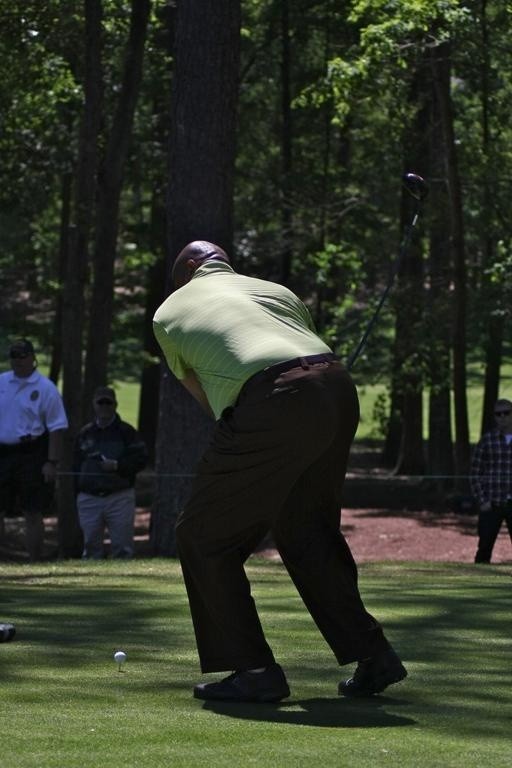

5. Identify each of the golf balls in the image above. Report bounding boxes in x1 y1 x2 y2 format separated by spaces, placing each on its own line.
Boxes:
115 651 127 662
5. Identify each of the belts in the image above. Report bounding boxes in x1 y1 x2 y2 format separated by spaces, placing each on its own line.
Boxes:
249 352 335 392
86 489 119 496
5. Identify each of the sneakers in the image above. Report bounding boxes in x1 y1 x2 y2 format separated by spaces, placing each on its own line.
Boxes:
336 648 407 697
194 661 289 704
32 550 57 560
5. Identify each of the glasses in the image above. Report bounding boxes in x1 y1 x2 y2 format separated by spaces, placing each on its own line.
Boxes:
10 352 32 358
97 398 113 405
495 410 512 417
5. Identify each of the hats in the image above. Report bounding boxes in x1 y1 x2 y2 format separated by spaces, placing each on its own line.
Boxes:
94 388 115 401
8 338 33 352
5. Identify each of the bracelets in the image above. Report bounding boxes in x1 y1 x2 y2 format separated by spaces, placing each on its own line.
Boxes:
48 459 63 469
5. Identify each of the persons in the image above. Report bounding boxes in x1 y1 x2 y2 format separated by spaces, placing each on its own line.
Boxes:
1 338 70 562
72 387 149 560
469 399 512 563
152 240 407 705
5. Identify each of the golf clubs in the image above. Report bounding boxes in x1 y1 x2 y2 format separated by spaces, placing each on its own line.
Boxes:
347 173 429 369
